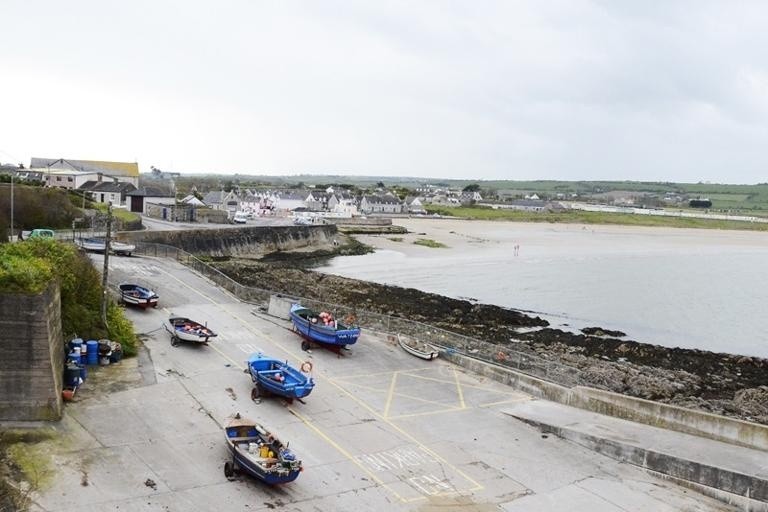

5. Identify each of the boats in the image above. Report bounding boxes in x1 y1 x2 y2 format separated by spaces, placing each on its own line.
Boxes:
247 349 317 405
167 311 217 347
289 303 362 354
224 412 302 484
107 239 137 255
397 332 439 360
74 237 109 252
118 280 159 308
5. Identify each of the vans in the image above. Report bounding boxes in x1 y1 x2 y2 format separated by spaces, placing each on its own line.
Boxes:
294 216 312 225
26 229 55 242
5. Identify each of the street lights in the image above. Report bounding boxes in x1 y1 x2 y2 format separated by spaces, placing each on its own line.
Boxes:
82 190 92 228
11 174 21 241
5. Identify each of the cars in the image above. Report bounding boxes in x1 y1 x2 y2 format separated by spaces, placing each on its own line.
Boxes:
233 210 247 224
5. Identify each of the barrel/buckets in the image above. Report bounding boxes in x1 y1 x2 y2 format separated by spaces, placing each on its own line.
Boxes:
87 341 98 364
69 353 81 365
72 339 83 348
259 446 269 458
8 236 18 242
68 368 80 389
78 365 87 381
249 443 258 454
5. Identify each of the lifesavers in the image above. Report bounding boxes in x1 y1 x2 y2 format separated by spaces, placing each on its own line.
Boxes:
301 361 312 373
